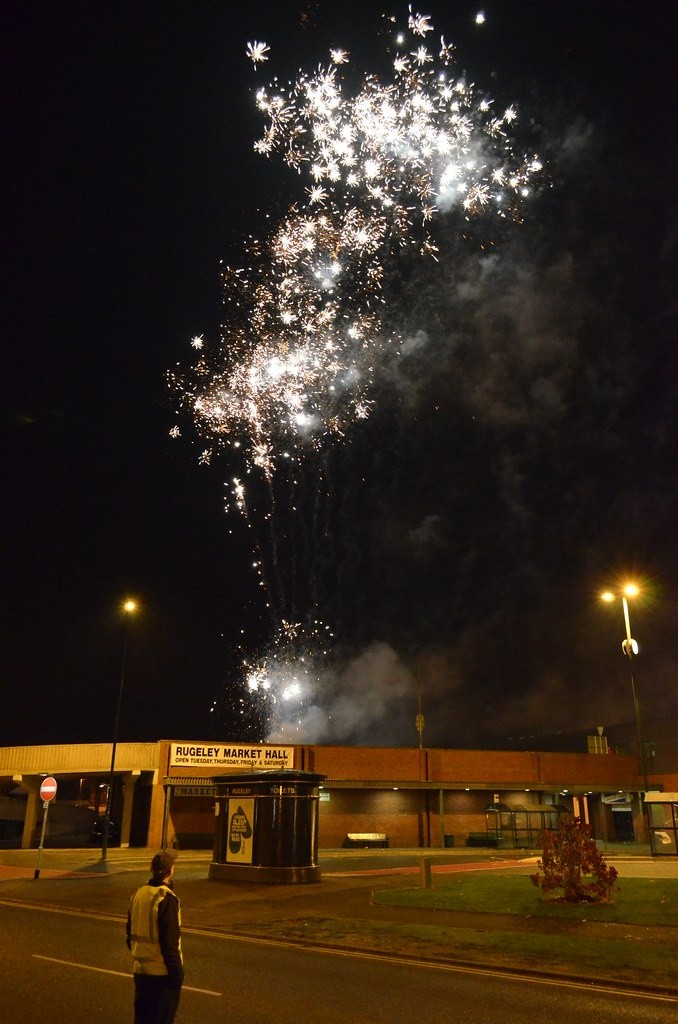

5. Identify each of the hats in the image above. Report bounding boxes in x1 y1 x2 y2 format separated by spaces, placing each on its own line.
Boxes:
151 849 181 872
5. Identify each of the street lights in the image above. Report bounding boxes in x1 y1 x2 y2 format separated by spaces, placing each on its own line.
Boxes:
101 599 138 859
601 582 650 794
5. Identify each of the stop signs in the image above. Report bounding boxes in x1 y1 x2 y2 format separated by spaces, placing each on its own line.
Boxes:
40 778 58 802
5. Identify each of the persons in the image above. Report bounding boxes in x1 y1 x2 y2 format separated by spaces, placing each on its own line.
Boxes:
126 849 184 1024
